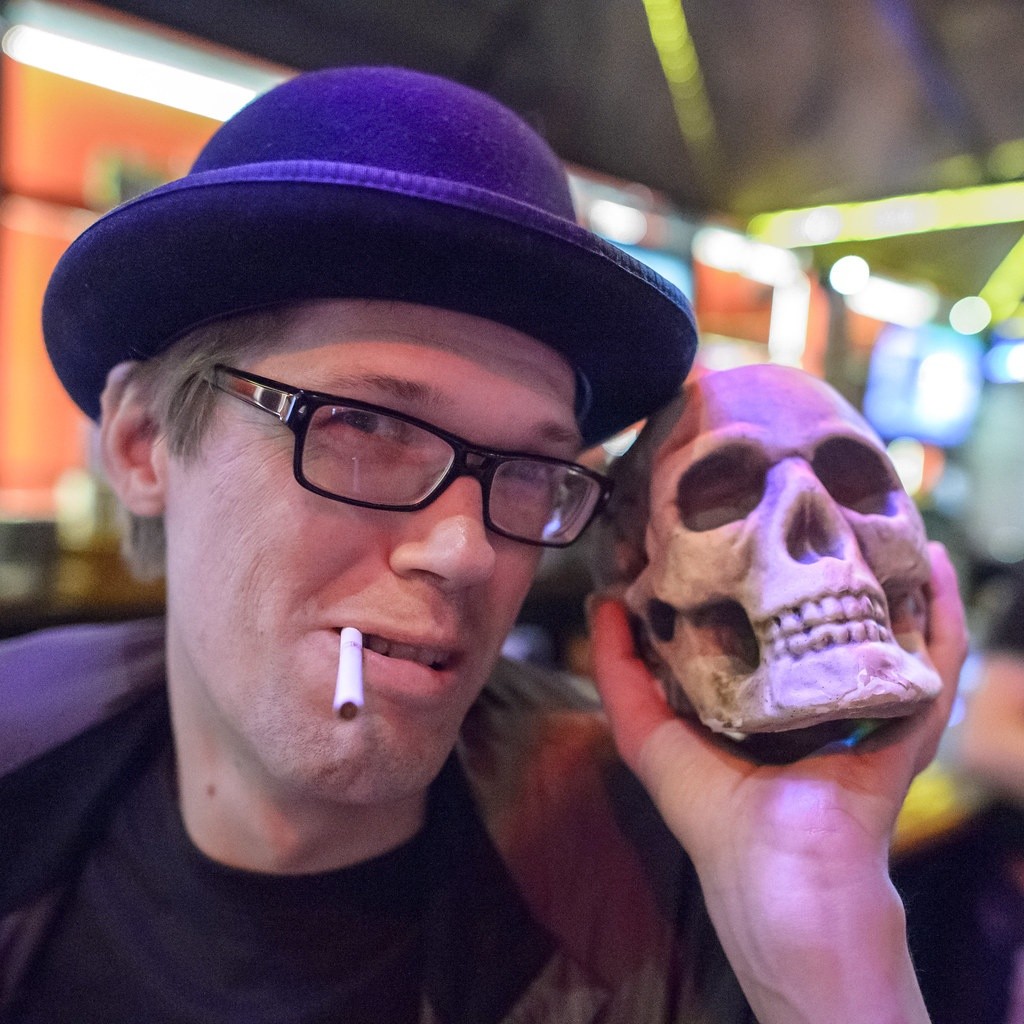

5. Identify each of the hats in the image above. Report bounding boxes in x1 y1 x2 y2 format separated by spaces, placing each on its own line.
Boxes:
40 66 698 457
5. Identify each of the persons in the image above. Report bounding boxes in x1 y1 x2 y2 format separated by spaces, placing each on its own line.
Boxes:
949 598 1024 793
0 71 976 1024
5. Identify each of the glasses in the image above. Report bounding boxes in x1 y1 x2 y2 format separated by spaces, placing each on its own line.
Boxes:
188 363 615 550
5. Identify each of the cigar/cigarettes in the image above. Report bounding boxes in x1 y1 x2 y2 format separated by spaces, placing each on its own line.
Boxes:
326 621 369 723
